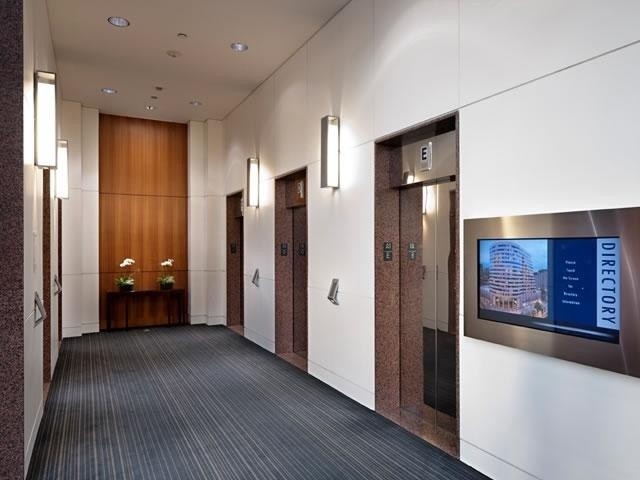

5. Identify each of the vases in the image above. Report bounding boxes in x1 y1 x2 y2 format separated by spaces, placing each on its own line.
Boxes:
161 283 173 289
120 285 132 291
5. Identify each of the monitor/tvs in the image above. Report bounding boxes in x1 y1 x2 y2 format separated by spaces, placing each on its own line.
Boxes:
464 207 640 379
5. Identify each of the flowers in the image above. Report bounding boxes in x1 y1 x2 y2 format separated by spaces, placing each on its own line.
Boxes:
114 258 135 287
156 258 176 285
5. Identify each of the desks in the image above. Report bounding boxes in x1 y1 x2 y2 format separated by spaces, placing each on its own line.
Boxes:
106 288 185 332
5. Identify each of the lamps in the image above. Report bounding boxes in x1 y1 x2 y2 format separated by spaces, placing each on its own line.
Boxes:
35 70 57 169
247 158 258 207
321 116 339 188
55 139 69 198
